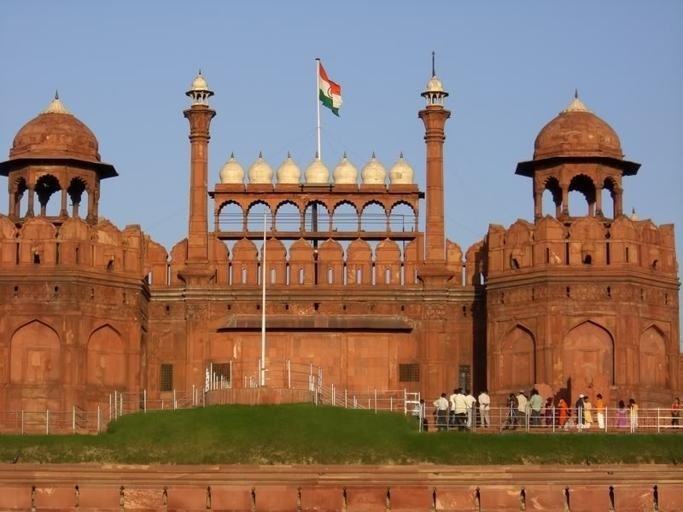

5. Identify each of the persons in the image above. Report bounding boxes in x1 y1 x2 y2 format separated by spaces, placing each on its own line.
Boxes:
671 397 680 429
413 387 639 432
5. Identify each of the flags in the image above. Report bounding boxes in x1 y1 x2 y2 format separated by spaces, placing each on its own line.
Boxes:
318 60 343 118
